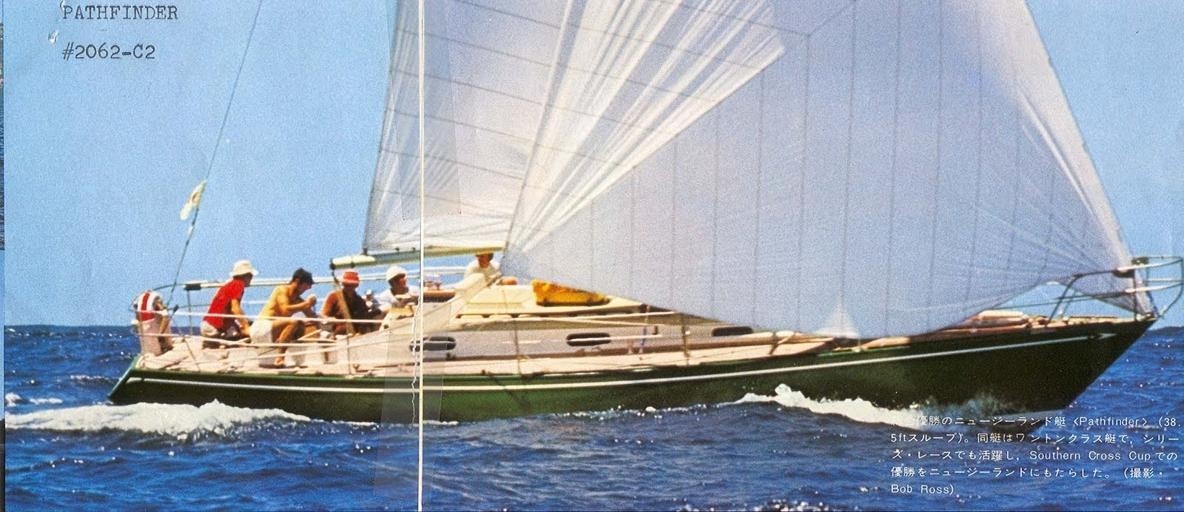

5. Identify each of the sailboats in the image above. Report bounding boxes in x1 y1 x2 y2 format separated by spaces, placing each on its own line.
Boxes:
106 1 1182 433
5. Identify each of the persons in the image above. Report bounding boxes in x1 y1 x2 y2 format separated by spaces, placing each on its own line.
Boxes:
370 265 422 312
462 251 501 281
200 259 256 347
317 270 372 334
248 266 322 364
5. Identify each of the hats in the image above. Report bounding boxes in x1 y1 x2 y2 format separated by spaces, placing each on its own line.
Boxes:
229 259 258 277
386 266 406 281
341 271 359 286
